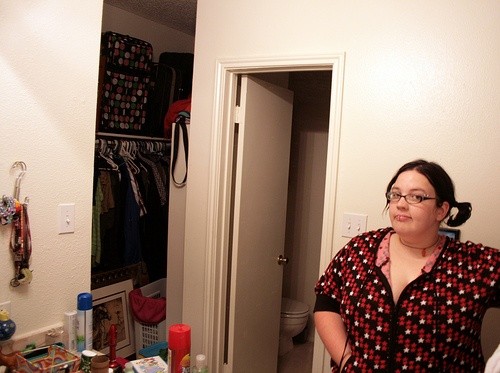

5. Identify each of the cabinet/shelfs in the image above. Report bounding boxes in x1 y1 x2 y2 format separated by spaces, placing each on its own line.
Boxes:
166 120 190 342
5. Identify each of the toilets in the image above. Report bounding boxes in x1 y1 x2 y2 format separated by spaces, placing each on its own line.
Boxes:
278 298 309 358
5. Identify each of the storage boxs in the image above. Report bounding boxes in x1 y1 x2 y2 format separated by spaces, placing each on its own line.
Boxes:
130 277 166 360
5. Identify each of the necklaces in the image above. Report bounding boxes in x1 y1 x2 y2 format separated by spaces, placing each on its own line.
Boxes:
399 236 440 257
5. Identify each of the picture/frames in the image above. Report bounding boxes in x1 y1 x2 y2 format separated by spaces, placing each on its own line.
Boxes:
91 279 135 358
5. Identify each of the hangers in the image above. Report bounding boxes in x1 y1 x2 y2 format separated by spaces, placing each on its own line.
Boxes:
96 140 167 171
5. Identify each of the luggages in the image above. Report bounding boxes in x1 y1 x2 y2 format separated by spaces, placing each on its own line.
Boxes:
152 52 195 137
95 31 154 135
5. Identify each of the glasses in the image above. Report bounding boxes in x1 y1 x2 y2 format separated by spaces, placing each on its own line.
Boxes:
386 192 440 205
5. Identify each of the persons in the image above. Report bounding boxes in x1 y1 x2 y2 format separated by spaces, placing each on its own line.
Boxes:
313 159 500 373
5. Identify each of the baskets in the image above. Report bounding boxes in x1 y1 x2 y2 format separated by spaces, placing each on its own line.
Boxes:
134 318 167 354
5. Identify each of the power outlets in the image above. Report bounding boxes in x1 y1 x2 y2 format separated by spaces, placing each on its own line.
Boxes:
0 301 14 318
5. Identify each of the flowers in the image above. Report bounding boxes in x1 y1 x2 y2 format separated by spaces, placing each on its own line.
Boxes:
1 196 21 227
